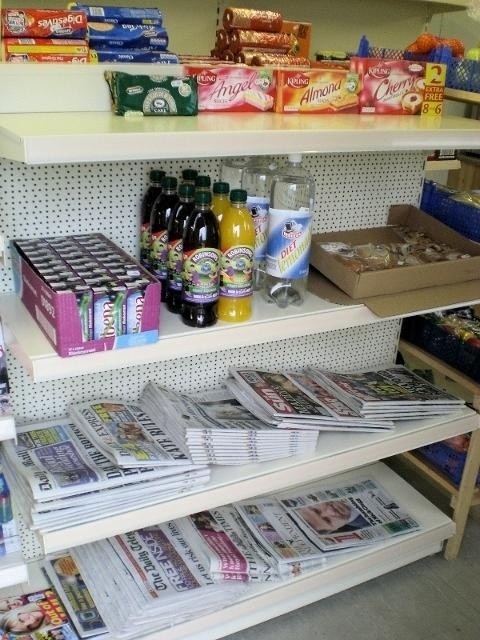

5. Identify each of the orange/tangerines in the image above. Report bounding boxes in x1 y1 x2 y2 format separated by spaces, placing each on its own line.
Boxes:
406 33 464 59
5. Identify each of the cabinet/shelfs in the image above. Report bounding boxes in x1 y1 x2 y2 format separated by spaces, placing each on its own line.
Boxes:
384 88 479 562
0 113 480 640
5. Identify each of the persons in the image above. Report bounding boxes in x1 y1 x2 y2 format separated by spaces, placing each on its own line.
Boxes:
296 500 372 534
1 604 47 636
1 597 24 616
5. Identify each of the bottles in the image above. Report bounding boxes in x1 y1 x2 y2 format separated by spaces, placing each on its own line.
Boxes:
138 153 315 326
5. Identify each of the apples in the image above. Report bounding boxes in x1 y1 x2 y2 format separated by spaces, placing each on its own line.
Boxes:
457 47 480 82
